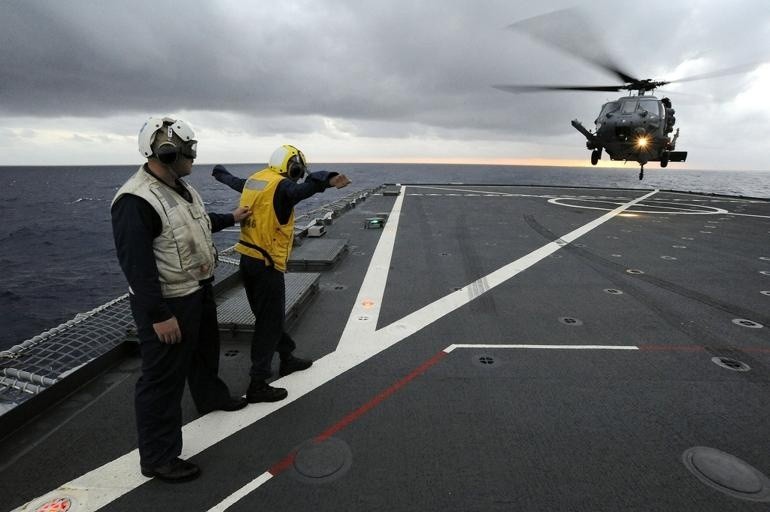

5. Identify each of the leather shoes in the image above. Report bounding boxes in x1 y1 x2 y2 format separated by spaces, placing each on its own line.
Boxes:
140 457 199 483
214 396 248 411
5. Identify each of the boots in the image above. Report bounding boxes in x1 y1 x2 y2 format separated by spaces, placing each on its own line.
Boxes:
280 352 312 376
247 374 287 403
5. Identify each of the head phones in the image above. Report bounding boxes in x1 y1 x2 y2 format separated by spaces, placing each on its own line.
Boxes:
156 126 181 165
288 153 304 181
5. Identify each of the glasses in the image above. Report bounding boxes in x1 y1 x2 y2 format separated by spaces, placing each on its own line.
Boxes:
152 140 197 157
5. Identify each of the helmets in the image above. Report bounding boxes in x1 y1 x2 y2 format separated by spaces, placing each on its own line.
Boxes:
138 115 195 157
268 145 307 173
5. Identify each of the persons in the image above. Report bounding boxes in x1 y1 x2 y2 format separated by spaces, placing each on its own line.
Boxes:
210 144 353 406
109 114 256 482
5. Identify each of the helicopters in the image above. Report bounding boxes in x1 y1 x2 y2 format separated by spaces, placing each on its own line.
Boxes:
477 3 770 181
